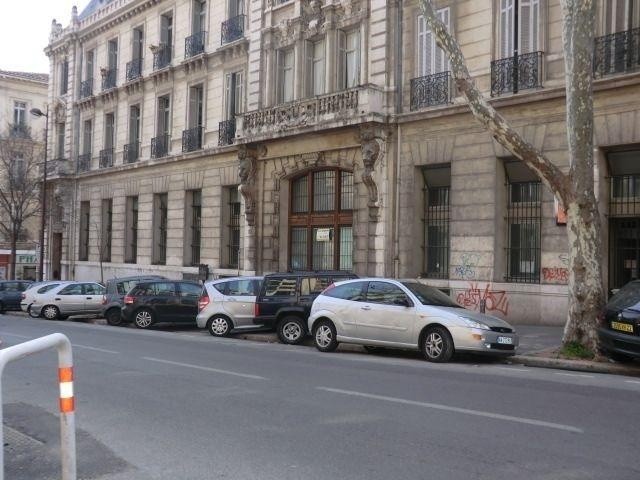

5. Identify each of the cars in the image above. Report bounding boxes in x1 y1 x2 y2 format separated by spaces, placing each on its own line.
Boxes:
593 279 640 365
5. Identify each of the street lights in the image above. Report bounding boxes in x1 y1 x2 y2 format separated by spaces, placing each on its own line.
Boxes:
29 105 49 284
